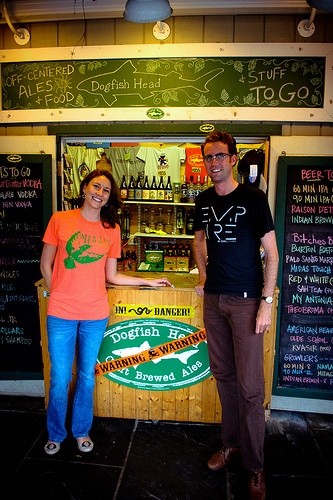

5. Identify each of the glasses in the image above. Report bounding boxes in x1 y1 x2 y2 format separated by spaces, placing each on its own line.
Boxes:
203 153 231 163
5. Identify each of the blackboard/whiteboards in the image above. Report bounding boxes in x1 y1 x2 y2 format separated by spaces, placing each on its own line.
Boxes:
0 41 333 124
273 157 332 401
0 152 53 381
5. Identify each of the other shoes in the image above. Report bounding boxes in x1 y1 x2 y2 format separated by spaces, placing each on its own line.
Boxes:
78 439 94 452
45 443 60 455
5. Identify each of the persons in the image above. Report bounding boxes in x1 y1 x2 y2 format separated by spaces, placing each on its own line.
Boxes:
192 132 280 500
40 169 171 455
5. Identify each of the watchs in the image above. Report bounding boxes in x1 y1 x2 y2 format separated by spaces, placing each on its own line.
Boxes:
261 296 273 303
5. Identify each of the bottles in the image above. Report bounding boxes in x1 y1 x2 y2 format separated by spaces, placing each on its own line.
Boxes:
119 175 215 204
117 207 195 272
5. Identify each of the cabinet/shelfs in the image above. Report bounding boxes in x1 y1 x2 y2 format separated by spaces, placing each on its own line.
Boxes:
122 200 196 239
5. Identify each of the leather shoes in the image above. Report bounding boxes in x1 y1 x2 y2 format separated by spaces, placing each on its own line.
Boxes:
208 446 236 469
249 473 266 500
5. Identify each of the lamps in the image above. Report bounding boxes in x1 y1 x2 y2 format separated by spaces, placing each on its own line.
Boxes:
298 0 333 37
123 0 173 40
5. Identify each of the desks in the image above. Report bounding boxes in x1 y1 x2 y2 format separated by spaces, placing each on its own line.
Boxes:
35 270 280 424
